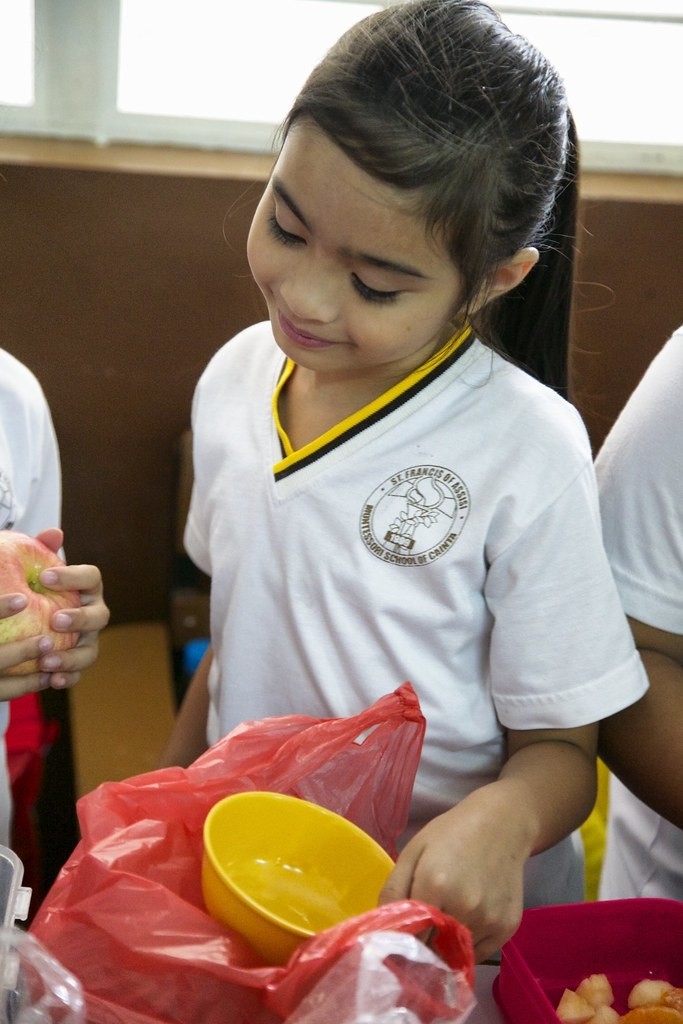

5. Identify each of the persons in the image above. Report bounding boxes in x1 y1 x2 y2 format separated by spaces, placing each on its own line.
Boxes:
161 0 650 961
594 327 683 903
0 348 110 843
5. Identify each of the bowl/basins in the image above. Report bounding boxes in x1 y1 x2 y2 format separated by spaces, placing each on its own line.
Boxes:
200 792 396 965
491 897 682 1024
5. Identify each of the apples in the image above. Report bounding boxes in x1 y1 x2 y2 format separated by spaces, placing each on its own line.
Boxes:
556 973 683 1024
0 530 82 677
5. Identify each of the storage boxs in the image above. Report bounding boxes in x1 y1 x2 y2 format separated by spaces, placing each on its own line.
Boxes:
67 622 179 800
493 896 683 1024
